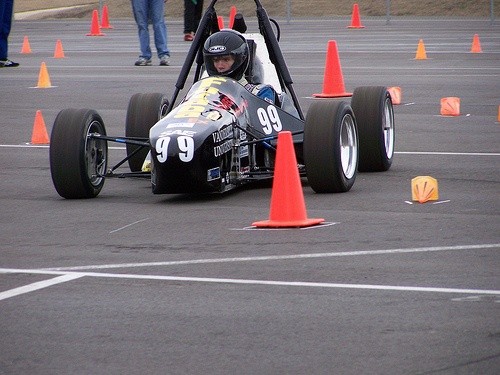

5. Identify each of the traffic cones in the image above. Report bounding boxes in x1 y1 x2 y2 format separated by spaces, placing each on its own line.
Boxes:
308 39 351 98
411 176 438 203
468 33 483 54
248 130 325 226
35 62 51 88
20 35 32 55
346 3 366 29
413 38 429 59
84 9 106 36
98 4 113 29
52 39 65 58
29 110 51 144
386 86 402 106
439 96 461 118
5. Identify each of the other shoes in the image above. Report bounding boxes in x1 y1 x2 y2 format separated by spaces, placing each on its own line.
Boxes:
184 33 193 41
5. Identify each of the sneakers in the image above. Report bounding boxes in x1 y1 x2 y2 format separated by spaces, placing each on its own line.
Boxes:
134 57 153 66
159 54 172 66
0 59 20 68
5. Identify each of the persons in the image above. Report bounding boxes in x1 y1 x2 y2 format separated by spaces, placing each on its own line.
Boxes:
203 30 274 104
0 0 21 68
183 0 203 41
130 0 171 66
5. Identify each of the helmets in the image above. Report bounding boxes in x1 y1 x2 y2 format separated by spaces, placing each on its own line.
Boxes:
203 28 250 81
